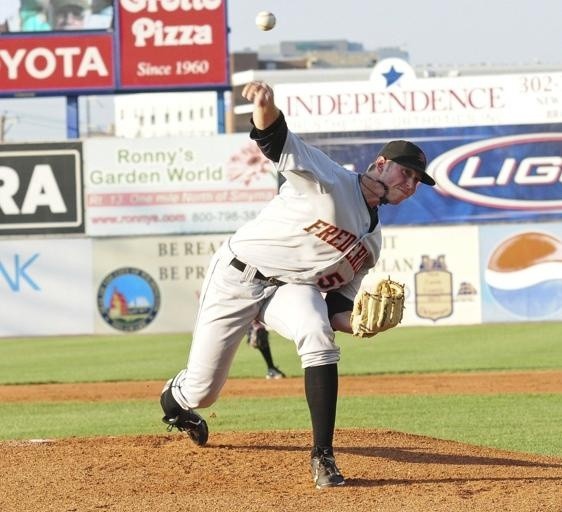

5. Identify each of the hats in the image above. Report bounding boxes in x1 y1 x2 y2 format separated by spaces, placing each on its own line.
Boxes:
378 141 436 186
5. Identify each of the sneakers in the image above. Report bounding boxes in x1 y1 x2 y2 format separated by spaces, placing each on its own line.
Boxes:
266 368 282 380
162 413 208 445
311 447 345 488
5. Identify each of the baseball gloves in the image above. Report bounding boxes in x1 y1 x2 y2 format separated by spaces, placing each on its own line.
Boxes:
350 276 405 339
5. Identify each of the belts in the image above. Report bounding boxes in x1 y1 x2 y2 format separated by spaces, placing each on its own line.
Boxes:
231 259 286 284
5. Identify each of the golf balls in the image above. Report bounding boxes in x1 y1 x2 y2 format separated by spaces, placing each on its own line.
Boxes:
256 12 275 31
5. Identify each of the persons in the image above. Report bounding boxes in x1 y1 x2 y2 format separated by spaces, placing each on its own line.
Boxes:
246 321 286 380
159 80 436 488
